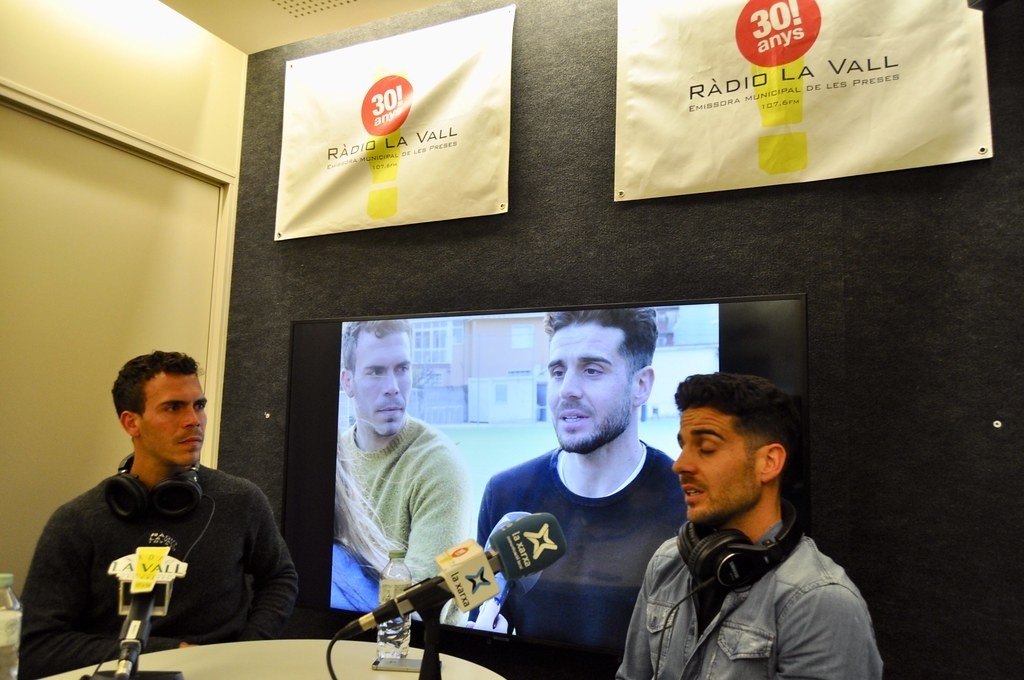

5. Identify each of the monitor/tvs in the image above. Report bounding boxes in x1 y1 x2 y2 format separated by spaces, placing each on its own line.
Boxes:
280 291 810 657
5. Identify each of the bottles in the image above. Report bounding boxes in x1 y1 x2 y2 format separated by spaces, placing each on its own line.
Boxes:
0 572 23 680
376 549 413 658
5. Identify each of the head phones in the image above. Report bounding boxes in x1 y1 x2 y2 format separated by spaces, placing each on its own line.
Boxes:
678 496 802 591
105 451 203 525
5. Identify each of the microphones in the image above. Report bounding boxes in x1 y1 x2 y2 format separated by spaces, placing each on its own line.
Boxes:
106 544 188 680
465 511 544 628
337 512 566 642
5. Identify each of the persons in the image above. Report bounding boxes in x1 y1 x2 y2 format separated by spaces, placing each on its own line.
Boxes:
329 318 474 628
16 352 295 680
615 372 885 680
330 457 404 613
472 307 690 651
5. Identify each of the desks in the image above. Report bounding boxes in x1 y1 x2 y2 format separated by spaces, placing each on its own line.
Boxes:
37 637 507 680
